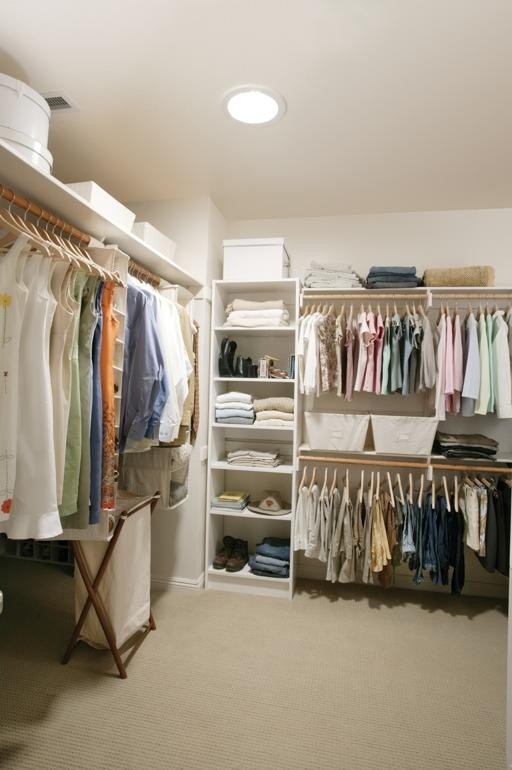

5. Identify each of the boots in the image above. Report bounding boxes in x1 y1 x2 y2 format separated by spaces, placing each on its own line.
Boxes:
218 338 258 379
423 265 495 288
212 534 234 569
225 538 250 574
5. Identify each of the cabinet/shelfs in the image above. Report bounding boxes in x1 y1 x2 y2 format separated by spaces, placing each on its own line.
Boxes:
1 534 75 568
204 278 302 597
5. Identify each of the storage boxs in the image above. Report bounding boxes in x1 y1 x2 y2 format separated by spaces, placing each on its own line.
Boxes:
136 223 181 262
66 179 136 231
220 238 292 280
0 72 57 176
303 408 441 461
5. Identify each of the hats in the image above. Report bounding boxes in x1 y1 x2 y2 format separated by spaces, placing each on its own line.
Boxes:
247 485 292 516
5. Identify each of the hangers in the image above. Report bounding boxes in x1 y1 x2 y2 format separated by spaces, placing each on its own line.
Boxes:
0 185 126 304
299 295 512 337
127 258 199 325
298 460 511 514
